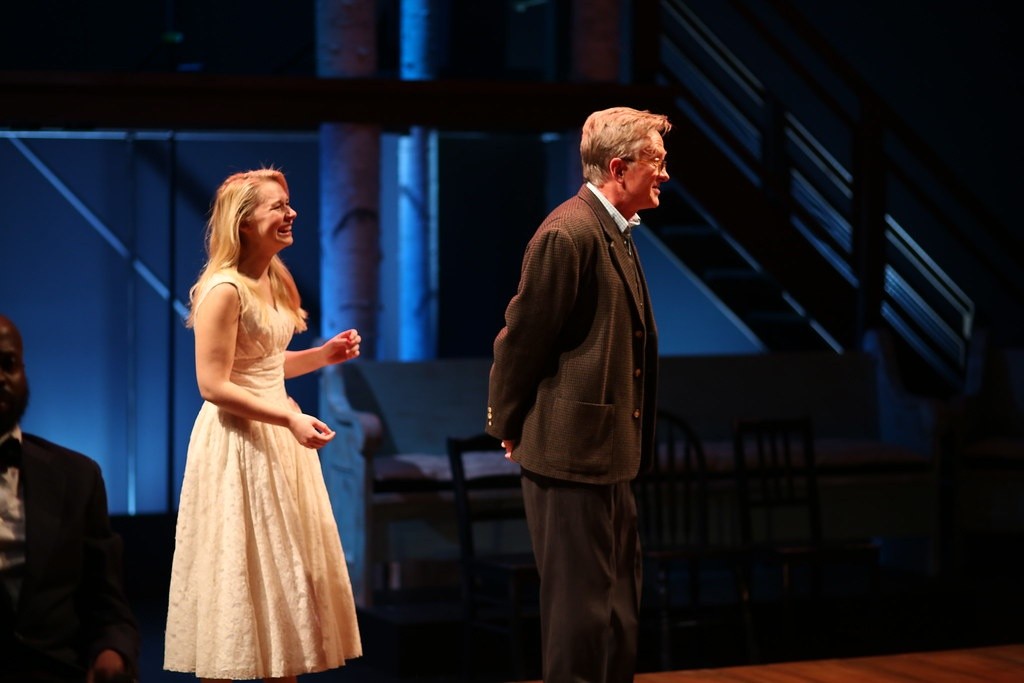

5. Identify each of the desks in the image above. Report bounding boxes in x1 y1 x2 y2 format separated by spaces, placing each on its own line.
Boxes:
633 645 1024 683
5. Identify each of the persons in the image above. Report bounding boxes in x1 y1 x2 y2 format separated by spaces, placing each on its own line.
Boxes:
163 169 365 683
486 107 673 683
0 314 145 683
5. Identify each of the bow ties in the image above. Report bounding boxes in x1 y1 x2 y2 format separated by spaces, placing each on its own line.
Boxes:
0 437 24 473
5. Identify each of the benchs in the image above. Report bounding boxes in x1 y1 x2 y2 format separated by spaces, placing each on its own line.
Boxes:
314 328 955 608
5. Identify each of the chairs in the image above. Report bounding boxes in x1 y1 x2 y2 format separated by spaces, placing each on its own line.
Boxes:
444 406 884 683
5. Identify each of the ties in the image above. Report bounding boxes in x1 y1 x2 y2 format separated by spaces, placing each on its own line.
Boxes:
622 226 633 258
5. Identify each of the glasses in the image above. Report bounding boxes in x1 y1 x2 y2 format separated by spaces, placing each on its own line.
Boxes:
624 153 667 170
